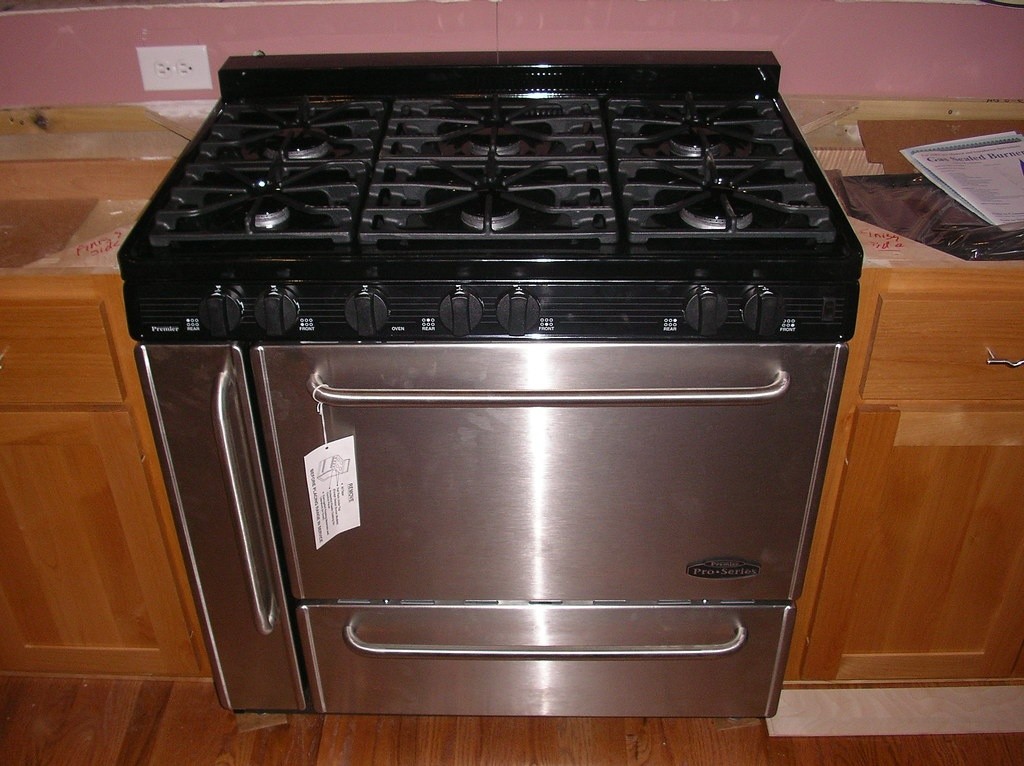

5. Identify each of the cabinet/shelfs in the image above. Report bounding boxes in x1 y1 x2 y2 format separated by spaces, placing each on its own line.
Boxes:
0 281 215 682
779 264 1024 688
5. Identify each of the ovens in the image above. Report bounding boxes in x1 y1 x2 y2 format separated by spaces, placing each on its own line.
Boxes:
133 342 850 718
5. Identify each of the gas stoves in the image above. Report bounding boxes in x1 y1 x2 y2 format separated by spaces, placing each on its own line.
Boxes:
116 50 864 343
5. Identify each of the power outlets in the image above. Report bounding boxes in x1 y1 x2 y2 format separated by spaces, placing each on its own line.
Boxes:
136 45 212 92
